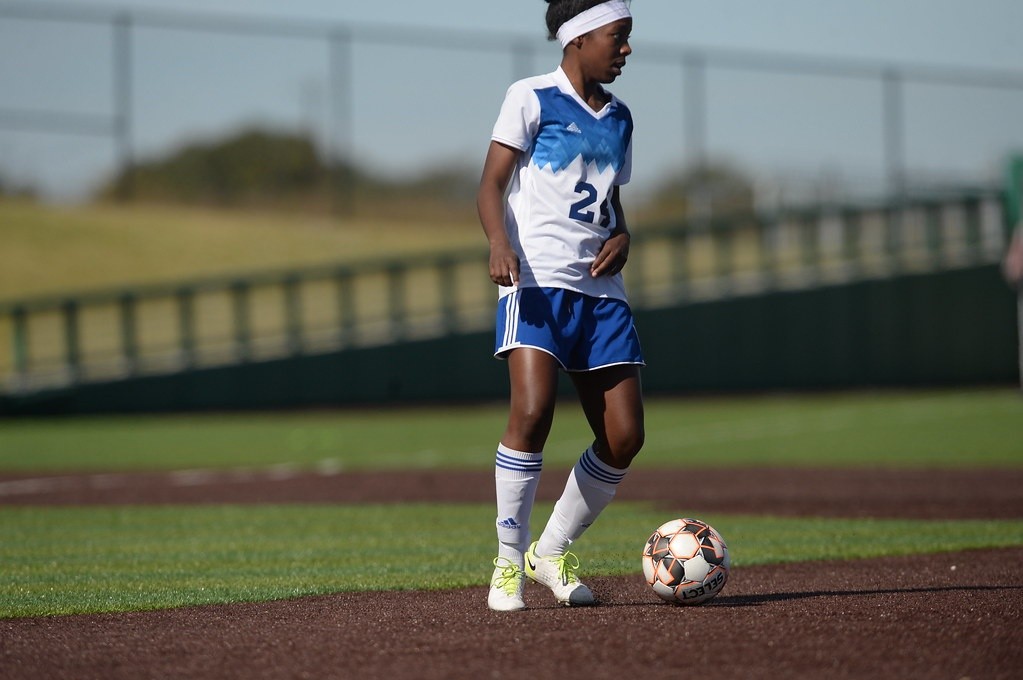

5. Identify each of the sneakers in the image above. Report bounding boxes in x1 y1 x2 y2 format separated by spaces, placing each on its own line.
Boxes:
524 541 593 606
488 557 525 611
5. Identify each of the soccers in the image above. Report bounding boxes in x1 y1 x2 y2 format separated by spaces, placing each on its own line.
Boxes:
642 518 729 605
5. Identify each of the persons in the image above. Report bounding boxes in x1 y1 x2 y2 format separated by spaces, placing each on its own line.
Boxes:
477 0 646 612
